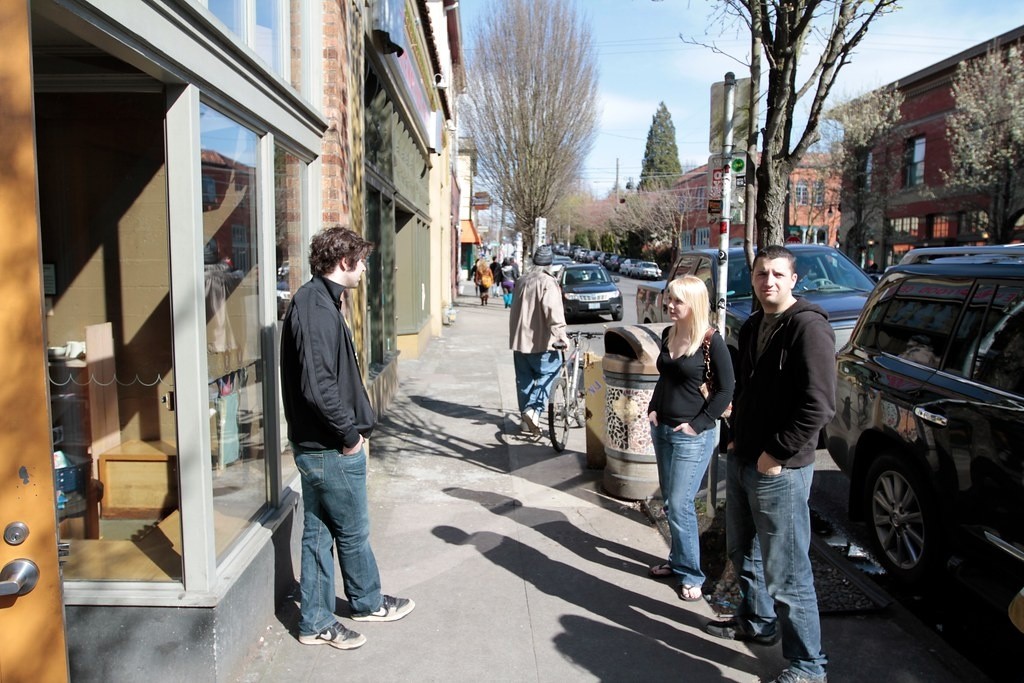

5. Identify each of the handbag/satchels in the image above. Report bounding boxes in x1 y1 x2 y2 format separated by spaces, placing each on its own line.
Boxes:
481 274 492 288
700 328 733 418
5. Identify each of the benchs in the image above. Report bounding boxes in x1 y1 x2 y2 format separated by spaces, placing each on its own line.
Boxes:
98 439 179 520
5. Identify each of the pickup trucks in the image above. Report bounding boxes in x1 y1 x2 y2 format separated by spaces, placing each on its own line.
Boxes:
636 244 877 396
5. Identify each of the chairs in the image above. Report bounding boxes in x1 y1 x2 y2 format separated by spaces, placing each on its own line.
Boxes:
566 274 575 283
591 272 597 279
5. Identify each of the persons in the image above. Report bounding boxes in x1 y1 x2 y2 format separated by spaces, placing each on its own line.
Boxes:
706 245 836 683
647 276 735 603
281 227 416 649
470 254 519 309
508 244 570 435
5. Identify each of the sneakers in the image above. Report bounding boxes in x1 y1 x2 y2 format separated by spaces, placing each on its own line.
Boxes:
520 421 530 432
298 621 367 650
706 617 781 647
522 406 542 434
350 593 416 623
768 668 828 683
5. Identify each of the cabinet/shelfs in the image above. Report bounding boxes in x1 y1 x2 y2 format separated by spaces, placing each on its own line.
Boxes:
47 322 120 458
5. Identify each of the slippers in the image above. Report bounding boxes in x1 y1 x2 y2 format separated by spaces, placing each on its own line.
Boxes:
681 585 703 601
648 563 674 579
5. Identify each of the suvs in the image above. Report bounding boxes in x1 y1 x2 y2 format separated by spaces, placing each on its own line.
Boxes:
820 243 1024 586
557 260 624 325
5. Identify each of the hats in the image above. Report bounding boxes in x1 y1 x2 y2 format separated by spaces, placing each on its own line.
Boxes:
533 245 553 266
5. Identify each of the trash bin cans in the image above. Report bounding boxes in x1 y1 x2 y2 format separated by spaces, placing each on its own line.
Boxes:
602 325 662 500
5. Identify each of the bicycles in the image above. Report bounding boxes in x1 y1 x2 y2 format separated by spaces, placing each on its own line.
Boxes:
548 330 603 453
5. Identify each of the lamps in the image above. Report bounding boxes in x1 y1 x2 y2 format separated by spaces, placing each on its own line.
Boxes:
432 72 447 90
827 201 842 218
443 119 456 132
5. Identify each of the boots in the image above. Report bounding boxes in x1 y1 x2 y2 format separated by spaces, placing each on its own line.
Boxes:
504 294 512 308
481 294 489 306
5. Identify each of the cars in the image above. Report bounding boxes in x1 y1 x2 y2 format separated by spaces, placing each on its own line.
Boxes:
597 253 612 266
630 261 662 281
552 243 601 264
604 255 627 272
551 255 576 277
619 259 642 276
